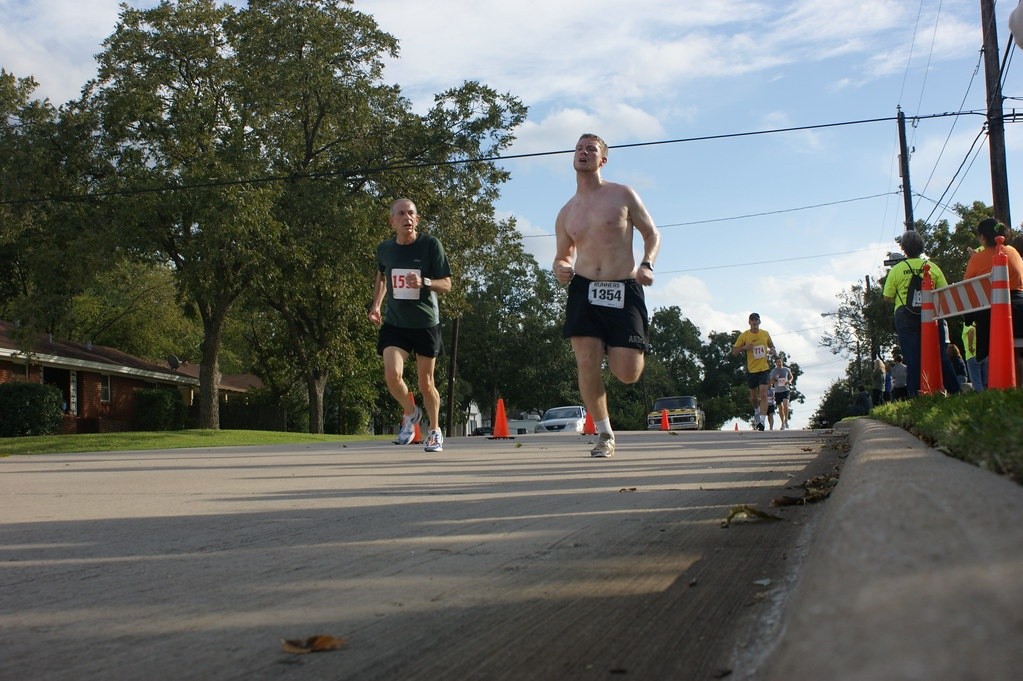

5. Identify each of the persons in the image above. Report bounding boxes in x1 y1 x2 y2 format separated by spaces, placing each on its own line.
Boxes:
369 199 452 452
552 133 661 458
732 313 793 432
853 218 1023 415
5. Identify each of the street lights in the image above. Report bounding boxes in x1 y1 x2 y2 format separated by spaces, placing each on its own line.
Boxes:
821 313 863 385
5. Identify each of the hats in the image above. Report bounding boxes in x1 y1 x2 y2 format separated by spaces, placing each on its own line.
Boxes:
749 313 759 319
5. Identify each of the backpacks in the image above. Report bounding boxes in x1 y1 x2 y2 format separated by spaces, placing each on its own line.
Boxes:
904 260 927 312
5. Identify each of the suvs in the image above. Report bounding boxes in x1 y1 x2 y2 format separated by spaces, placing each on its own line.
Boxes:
647 396 705 430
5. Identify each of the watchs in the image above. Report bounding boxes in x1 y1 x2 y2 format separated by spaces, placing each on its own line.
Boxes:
422 277 432 290
640 261 654 271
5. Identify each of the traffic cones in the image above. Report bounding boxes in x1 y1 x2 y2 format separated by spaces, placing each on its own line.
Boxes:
489 397 515 440
580 409 599 436
735 423 738 430
659 409 671 431
400 393 425 443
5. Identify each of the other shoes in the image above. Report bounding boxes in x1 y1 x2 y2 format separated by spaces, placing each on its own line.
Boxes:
754 415 765 431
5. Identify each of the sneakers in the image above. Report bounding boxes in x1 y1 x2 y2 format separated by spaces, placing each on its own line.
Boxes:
591 432 615 457
395 406 422 444
423 427 443 451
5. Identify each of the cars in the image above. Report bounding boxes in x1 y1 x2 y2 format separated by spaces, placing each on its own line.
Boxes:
467 425 493 436
534 405 588 433
